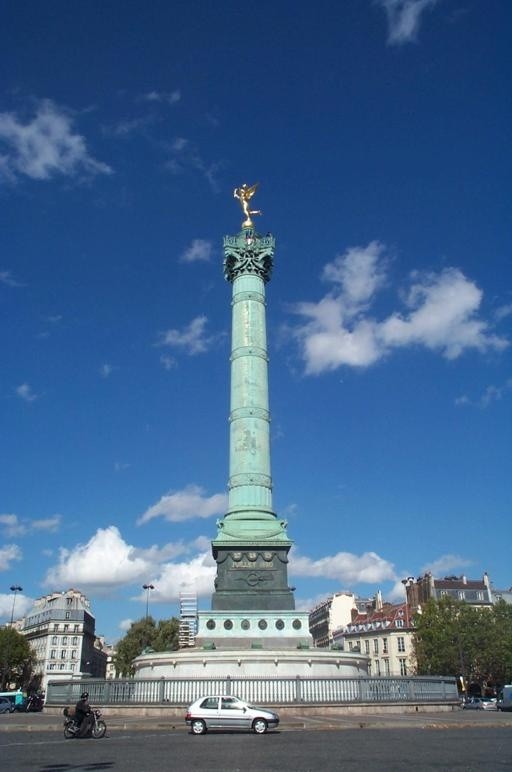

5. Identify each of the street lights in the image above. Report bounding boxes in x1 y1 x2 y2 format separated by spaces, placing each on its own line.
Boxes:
143 584 155 624
2 585 23 692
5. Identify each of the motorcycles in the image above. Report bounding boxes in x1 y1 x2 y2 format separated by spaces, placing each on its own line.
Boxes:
63 707 106 739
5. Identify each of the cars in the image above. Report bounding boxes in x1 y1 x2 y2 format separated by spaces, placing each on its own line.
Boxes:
185 695 279 735
0 691 44 713
460 685 512 712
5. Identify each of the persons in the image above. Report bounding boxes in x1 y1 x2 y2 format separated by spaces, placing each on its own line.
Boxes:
75 691 94 731
234 184 262 221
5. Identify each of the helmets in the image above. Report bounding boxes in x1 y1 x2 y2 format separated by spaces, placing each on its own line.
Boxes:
81 692 88 698
